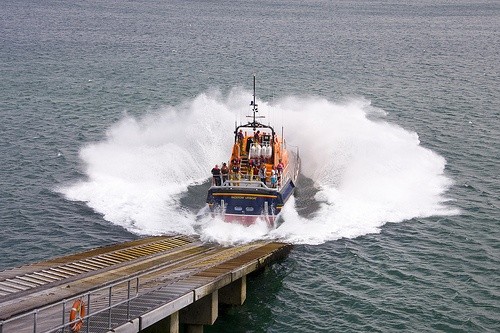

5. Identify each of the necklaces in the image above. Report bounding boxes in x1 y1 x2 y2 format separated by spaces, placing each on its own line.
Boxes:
231 155 240 178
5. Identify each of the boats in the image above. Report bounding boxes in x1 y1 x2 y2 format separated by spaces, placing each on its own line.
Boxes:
204 75 301 232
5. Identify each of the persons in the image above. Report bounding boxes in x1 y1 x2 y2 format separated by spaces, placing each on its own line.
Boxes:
255 130 260 143
221 163 231 186
271 159 285 188
237 130 244 145
245 155 264 179
273 132 278 144
211 164 221 186
260 165 267 187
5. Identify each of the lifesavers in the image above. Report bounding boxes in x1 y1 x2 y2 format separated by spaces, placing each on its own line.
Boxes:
69 300 86 333
